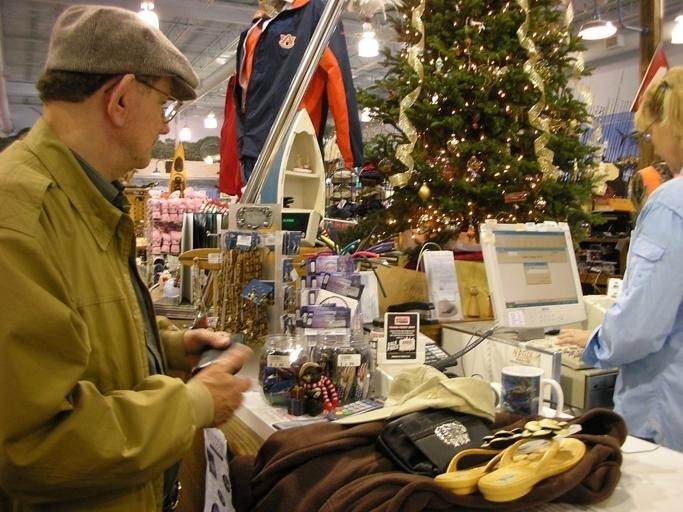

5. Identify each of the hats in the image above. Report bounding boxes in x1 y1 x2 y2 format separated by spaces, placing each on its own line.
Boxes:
43 3 200 100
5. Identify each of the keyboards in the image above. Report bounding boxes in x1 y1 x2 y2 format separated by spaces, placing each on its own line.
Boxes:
524 338 595 370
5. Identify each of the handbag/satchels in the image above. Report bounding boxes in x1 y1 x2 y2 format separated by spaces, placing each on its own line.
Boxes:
379 405 494 478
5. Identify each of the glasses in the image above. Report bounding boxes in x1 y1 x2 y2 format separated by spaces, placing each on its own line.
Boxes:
103 75 184 125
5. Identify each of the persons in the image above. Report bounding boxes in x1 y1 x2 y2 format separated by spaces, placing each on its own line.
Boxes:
555 66 683 454
0 4 251 512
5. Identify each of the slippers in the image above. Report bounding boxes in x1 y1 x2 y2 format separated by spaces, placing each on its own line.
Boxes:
435 448 503 495
478 435 586 502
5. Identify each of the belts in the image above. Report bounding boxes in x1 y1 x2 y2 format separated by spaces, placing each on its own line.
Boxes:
160 482 183 510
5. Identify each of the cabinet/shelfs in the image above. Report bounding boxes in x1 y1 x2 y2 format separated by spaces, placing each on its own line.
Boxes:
564 198 639 284
262 123 328 215
143 202 205 285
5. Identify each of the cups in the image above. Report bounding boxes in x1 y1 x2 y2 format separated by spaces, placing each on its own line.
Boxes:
502 365 564 421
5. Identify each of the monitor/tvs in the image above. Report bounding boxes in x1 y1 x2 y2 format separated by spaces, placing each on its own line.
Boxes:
281 208 320 247
479 223 587 345
